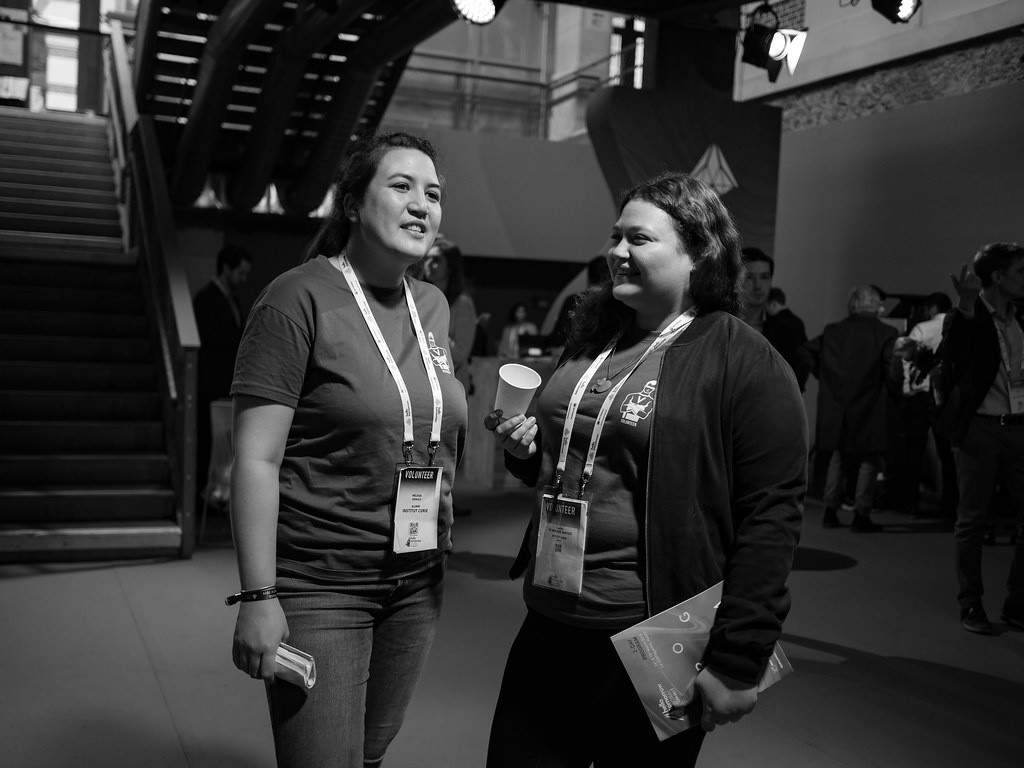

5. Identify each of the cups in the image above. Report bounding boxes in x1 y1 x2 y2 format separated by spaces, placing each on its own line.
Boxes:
492 363 541 421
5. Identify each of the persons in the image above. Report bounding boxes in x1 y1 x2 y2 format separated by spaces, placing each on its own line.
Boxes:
488 170 809 768
191 244 1024 634
219 133 471 768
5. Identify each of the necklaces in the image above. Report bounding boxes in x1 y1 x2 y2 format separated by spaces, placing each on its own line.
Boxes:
591 340 654 393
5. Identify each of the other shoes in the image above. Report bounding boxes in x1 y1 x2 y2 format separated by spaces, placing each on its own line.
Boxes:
983 530 995 545
961 605 992 633
851 512 884 533
822 505 840 526
806 484 824 502
1001 597 1024 626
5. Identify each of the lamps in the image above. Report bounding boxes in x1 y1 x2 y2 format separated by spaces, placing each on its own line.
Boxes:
452 1 508 26
740 0 808 83
870 0 921 25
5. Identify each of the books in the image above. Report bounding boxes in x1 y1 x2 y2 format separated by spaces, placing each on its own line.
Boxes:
274 642 317 689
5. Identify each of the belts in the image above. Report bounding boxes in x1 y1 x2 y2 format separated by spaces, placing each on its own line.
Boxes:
975 414 1024 425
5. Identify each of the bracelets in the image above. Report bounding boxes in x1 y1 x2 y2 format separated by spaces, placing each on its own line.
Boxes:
225 586 278 606
